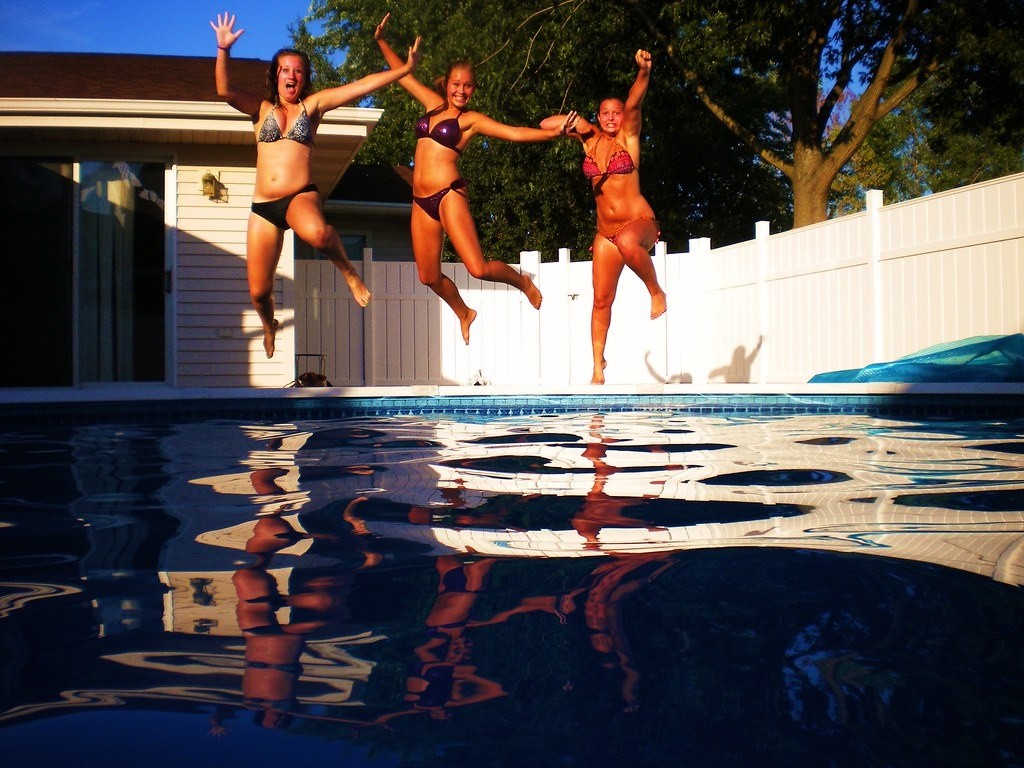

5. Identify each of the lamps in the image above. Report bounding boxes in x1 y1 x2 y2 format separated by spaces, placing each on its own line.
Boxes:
202 170 221 198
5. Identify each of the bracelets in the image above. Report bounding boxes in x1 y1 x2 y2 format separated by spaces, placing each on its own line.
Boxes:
217 45 231 50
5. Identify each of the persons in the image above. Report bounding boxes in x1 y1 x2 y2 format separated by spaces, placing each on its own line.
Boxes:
373 12 581 346
540 49 667 386
209 11 421 359
